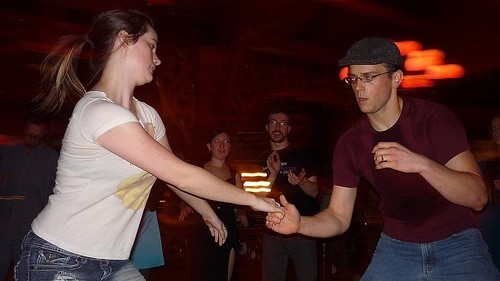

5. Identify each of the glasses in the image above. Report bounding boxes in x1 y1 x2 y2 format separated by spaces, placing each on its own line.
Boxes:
269 120 289 126
343 70 396 85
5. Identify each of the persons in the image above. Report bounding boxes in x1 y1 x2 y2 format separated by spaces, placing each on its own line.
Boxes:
0 112 58 281
13 9 286 281
178 129 248 281
481 114 500 207
266 38 500 281
251 109 318 281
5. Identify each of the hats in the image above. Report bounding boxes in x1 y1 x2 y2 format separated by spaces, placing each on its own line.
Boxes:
338 36 402 67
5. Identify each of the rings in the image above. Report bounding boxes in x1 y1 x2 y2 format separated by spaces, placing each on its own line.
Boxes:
381 154 385 160
